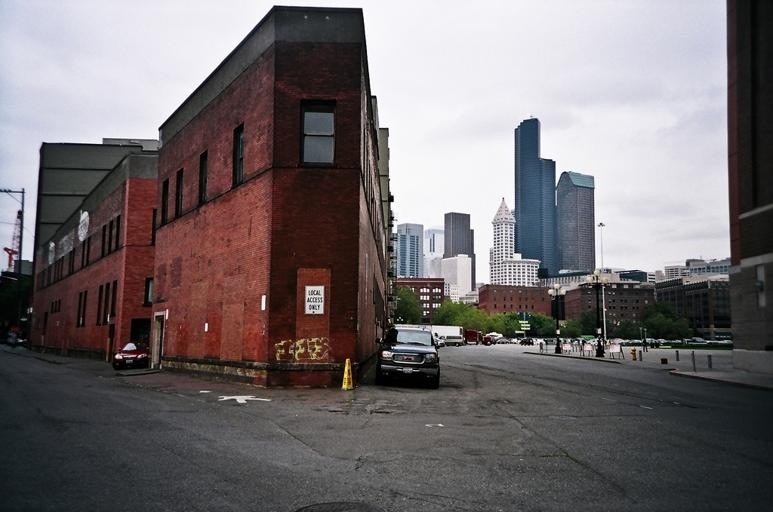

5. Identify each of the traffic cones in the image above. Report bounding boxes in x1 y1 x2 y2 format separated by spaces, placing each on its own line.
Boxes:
340 358 355 391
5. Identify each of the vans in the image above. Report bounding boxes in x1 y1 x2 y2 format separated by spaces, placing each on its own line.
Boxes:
439 335 463 346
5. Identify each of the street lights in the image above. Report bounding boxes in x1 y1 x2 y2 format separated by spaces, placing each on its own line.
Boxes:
548 284 566 352
587 270 608 357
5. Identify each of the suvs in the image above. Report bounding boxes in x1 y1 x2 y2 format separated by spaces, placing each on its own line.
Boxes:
375 327 440 390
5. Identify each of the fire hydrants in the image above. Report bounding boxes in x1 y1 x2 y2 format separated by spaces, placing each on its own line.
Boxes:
631 348 637 360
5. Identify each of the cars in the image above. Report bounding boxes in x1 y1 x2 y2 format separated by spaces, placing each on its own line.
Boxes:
573 338 603 345
627 337 667 344
497 338 533 346
112 341 150 370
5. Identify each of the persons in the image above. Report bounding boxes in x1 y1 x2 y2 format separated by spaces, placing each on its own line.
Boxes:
643 338 649 353
